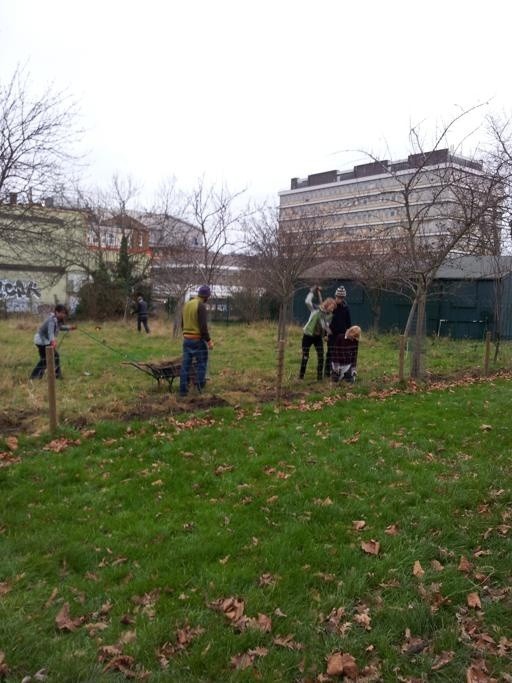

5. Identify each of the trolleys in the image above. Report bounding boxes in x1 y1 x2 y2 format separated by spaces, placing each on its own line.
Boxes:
122 358 211 390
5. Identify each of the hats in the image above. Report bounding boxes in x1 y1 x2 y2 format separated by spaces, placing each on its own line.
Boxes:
335 286 346 297
198 286 210 297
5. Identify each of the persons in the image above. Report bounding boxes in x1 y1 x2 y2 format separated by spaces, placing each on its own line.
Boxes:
31 304 77 382
180 285 214 398
297 286 361 389
130 296 152 334
